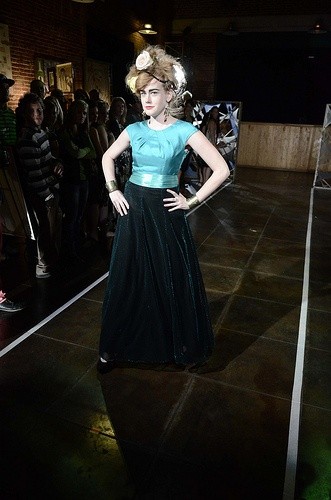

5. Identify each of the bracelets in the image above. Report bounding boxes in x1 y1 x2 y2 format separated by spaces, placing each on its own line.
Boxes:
186 194 201 210
105 180 119 193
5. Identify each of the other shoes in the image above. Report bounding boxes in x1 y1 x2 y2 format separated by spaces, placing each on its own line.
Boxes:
96 358 112 372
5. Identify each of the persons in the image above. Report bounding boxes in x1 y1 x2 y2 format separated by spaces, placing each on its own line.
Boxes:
196 114 211 182
97 44 231 375
202 106 219 185
0 73 150 312
180 99 196 183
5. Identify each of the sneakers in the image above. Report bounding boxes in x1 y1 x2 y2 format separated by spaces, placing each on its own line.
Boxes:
0 299 24 313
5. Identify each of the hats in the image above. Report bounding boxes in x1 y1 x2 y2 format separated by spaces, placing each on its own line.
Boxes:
0 74 15 86
